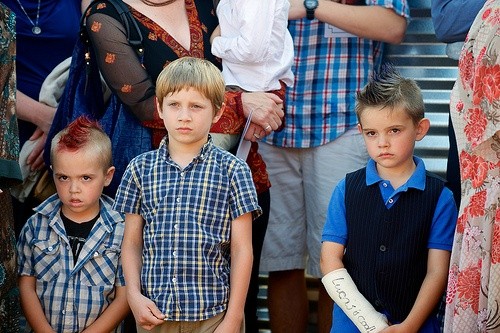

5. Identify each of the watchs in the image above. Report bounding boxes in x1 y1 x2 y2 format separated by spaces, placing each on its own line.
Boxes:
304 0 319 20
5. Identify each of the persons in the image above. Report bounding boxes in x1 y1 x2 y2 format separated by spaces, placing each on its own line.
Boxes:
0 0 500 333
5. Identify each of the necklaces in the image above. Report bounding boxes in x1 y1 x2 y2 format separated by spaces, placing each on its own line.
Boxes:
18 0 42 35
141 0 177 7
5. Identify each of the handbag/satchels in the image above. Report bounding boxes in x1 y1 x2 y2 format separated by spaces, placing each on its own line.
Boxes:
43 0 152 199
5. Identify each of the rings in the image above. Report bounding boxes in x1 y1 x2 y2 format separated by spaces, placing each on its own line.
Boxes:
264 125 270 130
254 133 260 138
